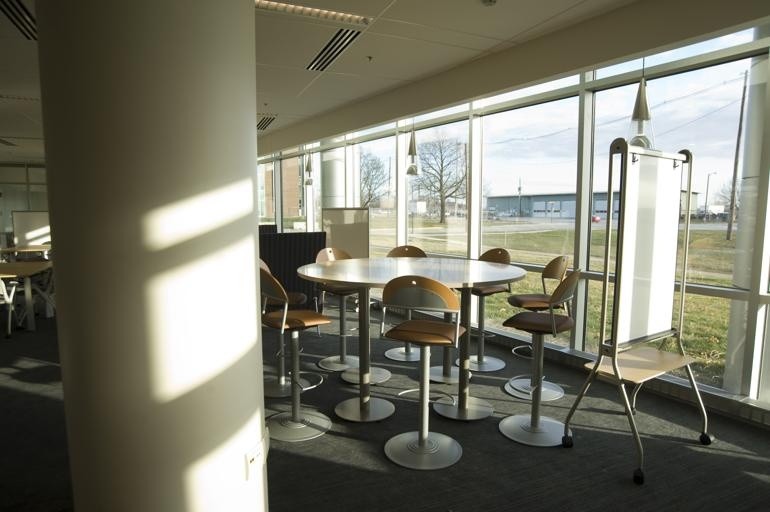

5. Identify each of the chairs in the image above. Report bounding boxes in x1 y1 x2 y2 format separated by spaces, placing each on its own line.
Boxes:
259 258 311 398
384 246 431 361
504 250 569 401
380 275 466 470
497 269 583 447
316 248 360 372
455 248 511 372
259 267 332 442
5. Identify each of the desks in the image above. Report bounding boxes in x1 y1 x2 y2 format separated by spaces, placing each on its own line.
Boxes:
0 241 56 339
297 257 527 421
